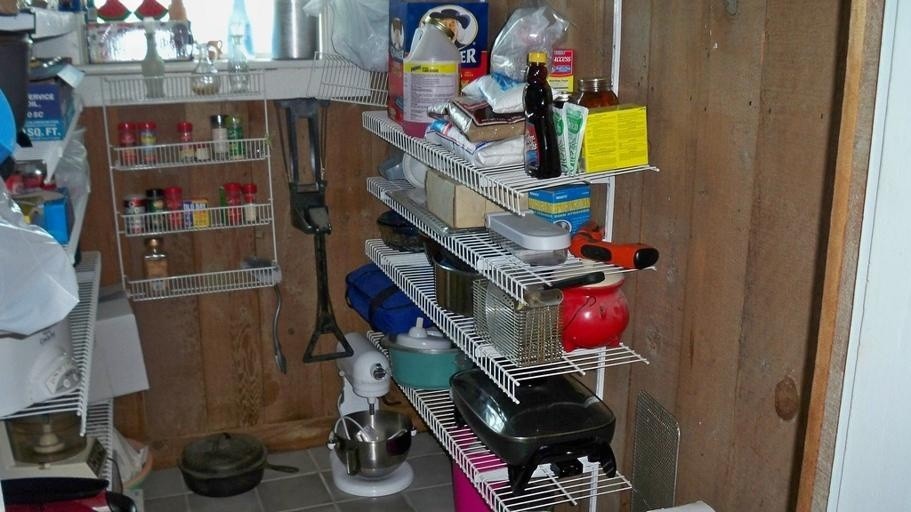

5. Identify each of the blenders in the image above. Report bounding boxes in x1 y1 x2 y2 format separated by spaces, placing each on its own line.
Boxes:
324 328 417 497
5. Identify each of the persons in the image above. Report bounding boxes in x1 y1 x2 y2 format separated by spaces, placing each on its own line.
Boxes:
391 17 405 49
431 7 469 49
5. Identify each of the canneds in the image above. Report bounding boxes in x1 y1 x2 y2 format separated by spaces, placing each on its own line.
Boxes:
118 115 259 234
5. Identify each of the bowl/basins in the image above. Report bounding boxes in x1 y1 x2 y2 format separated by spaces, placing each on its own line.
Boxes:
333 407 414 483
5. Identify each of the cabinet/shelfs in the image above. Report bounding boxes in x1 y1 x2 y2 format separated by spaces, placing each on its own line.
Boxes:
99 69 283 303
0 14 130 512
360 107 662 512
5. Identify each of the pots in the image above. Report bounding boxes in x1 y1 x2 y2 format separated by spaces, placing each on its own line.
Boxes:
175 430 301 499
446 365 618 498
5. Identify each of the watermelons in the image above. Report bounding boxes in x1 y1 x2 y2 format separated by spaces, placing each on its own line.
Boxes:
135 0 168 21
98 0 131 22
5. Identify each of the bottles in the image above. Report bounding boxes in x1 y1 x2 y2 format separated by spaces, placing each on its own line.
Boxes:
139 29 168 99
188 41 224 95
122 182 258 235
116 113 246 165
141 237 169 297
523 49 563 181
576 76 619 112
227 32 253 95
